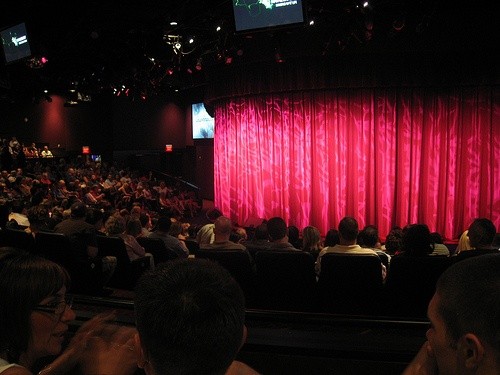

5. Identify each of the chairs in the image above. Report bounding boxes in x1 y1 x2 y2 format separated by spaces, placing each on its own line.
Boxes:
1 225 499 326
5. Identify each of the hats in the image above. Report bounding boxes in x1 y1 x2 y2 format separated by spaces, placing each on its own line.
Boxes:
87 208 104 222
71 202 89 216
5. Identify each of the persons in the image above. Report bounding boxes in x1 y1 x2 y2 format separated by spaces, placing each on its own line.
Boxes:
0 137 500 375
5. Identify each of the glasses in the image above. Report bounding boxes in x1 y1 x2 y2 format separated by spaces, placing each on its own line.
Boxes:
34 294 73 320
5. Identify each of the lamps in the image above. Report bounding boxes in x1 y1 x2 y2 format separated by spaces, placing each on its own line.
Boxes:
0 0 439 108
